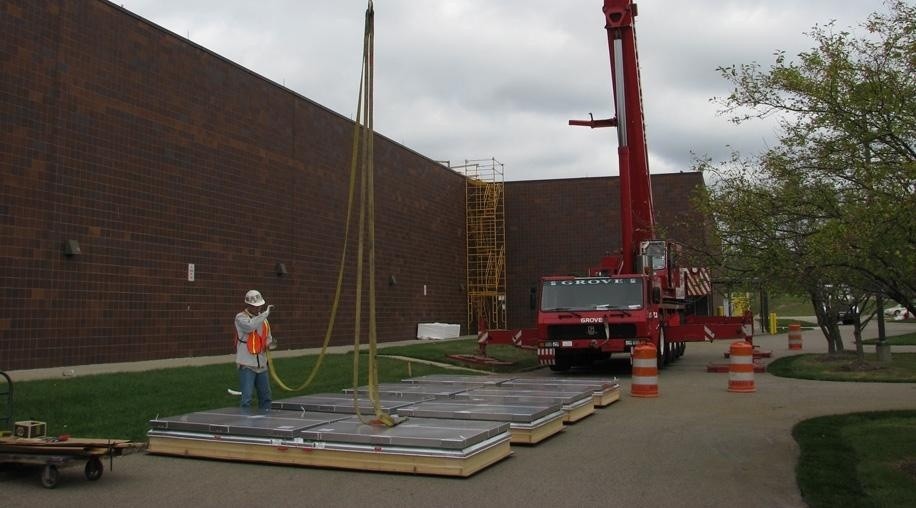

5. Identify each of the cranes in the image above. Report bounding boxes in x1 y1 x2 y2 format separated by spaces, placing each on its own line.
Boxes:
477 1 753 370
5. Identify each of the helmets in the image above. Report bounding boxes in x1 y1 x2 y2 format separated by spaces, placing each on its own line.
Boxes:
244 290 266 307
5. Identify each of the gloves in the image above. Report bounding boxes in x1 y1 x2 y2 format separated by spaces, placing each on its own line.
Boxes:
266 305 274 316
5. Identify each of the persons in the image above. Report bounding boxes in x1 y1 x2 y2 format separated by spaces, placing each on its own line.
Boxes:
234 289 275 412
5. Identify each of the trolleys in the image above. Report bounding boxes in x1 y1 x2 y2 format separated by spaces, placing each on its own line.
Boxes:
1 371 123 489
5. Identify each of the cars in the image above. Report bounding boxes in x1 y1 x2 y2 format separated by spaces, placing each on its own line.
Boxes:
884 304 910 316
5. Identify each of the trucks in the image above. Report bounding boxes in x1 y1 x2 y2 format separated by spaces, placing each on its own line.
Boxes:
823 284 860 324
